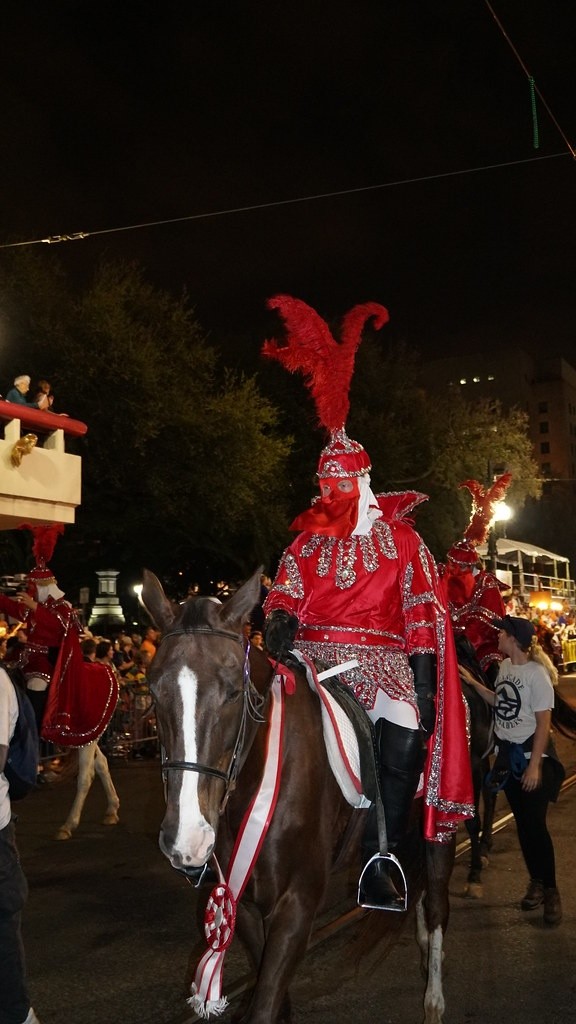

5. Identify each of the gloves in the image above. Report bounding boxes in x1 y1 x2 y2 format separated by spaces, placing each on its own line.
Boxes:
265 609 295 654
408 653 438 742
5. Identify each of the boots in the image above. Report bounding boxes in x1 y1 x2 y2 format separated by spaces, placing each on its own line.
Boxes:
357 718 425 910
178 864 217 884
26 687 48 739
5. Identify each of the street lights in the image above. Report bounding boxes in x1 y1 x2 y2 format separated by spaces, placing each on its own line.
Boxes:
497 501 512 538
459 373 498 575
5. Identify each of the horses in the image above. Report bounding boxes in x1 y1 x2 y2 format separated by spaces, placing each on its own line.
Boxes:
142 566 576 1024
3 662 120 840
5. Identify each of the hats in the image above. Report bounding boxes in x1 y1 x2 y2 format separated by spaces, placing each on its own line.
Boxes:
493 615 536 646
447 472 512 565
121 637 134 646
17 519 64 583
261 294 389 480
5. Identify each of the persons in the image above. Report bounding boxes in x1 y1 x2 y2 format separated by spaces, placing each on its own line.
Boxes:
4 375 69 440
437 540 514 714
454 611 563 926
0 565 119 748
504 588 576 676
0 620 263 763
258 441 476 904
0 667 40 1024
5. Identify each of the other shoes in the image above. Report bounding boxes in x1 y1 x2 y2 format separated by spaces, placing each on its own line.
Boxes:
521 879 543 909
543 887 562 924
